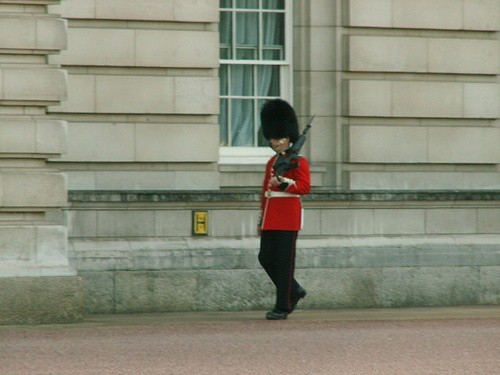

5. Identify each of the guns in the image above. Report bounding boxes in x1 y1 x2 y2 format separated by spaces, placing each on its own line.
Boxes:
270 113 316 183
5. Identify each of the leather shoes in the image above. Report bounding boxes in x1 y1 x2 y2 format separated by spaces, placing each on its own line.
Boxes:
288 286 307 314
265 311 288 319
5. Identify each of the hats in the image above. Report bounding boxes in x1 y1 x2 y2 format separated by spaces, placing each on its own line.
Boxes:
260 97 301 141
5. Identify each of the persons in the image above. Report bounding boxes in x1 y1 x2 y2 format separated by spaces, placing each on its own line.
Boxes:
256 126 311 321
